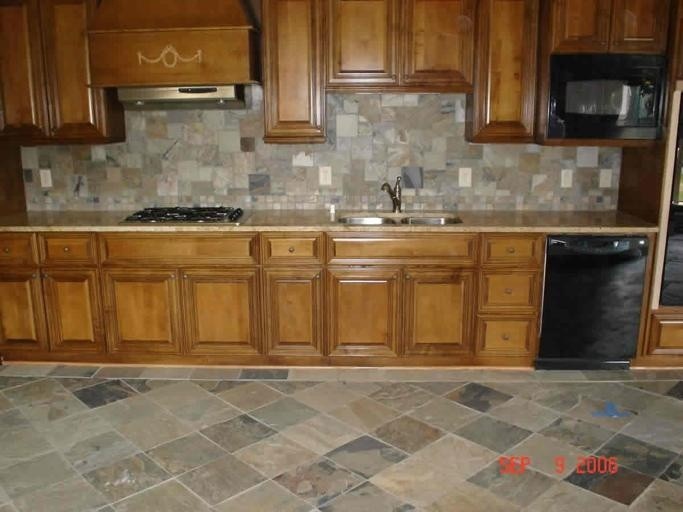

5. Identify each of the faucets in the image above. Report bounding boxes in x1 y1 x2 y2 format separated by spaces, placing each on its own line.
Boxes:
380 176 402 214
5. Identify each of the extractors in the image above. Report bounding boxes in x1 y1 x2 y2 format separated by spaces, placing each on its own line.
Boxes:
115 85 245 107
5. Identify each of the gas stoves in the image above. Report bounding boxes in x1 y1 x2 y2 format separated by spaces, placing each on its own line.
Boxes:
115 206 253 226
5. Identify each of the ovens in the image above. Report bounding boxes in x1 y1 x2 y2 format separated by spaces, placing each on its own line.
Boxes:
534 235 650 372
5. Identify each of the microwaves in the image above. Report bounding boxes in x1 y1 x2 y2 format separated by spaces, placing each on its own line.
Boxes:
546 49 662 143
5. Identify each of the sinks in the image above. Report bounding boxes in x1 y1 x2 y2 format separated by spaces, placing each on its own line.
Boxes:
399 214 465 225
336 215 398 225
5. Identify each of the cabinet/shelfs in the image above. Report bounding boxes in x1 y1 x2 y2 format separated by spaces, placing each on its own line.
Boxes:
326 231 481 370
530 0 670 58
0 0 124 146
314 0 475 94
256 0 327 144
95 231 263 369
464 1 537 143
0 231 105 368
473 231 549 370
263 230 328 366
